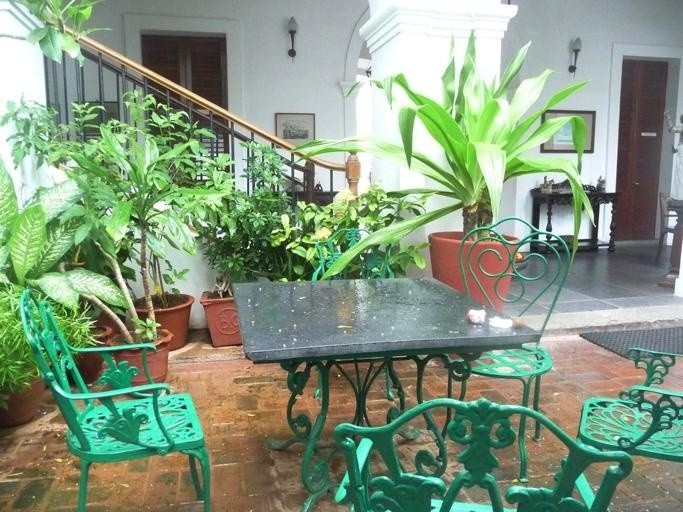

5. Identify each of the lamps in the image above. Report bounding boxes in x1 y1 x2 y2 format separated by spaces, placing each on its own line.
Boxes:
287 16 299 62
569 36 582 79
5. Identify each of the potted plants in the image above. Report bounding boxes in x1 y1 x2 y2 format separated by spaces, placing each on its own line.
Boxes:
0 87 226 425
196 139 252 347
290 28 597 311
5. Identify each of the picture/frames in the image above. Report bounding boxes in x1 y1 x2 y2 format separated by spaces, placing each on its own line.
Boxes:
275 112 315 148
540 109 596 153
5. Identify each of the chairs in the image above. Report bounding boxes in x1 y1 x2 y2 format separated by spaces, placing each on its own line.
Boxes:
457 218 571 483
19 290 211 512
332 397 632 512
654 191 678 262
579 347 683 462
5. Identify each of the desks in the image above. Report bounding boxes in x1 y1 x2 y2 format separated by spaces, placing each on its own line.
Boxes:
231 278 542 511
530 179 624 252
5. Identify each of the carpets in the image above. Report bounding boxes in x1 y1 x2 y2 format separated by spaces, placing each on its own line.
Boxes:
580 328 683 361
